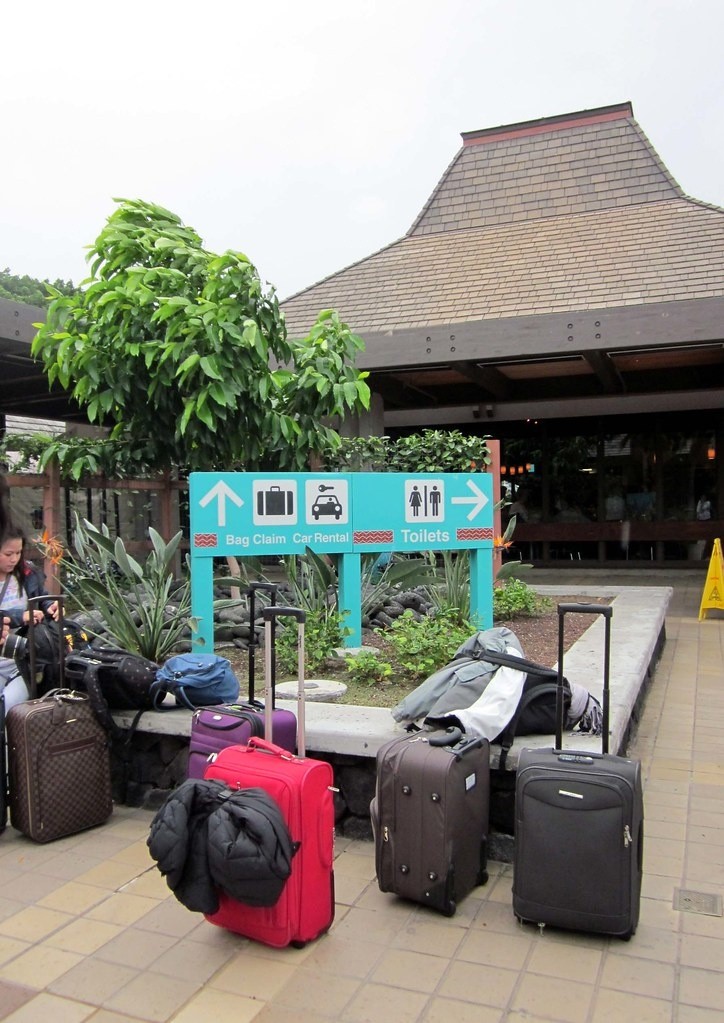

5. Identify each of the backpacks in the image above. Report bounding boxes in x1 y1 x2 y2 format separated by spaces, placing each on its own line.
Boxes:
149 654 241 710
65 647 167 744
13 619 89 700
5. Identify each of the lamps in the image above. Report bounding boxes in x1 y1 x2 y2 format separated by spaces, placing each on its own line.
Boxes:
578 460 593 472
473 404 494 418
708 446 716 460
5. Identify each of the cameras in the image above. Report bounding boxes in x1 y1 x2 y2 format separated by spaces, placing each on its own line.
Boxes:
0 633 28 660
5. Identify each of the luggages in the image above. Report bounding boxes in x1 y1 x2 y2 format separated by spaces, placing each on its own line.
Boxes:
190 583 298 780
511 602 643 941
369 726 490 915
7 594 114 842
203 607 334 947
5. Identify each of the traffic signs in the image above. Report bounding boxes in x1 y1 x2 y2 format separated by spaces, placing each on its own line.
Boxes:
349 471 496 552
189 472 352 554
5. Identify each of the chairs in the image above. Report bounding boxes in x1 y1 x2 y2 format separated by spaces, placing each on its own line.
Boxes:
505 512 658 562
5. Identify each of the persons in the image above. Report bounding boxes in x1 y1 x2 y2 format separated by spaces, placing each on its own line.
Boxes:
0 525 66 715
508 489 551 560
605 484 624 560
696 492 711 521
